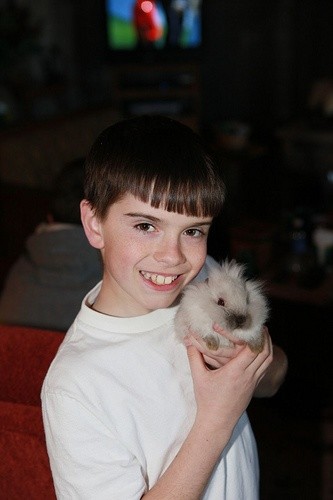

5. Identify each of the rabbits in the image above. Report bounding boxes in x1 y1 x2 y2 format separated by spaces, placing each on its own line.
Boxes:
170 256 271 356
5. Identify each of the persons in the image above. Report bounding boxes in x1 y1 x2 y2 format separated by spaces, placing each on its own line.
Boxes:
40 115 288 500
